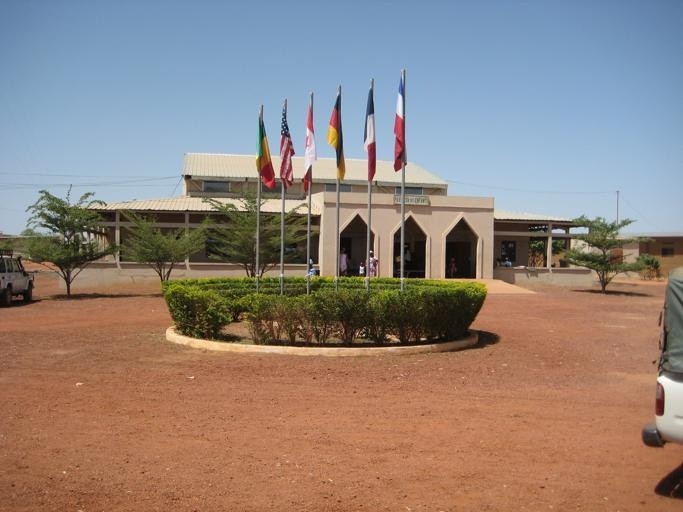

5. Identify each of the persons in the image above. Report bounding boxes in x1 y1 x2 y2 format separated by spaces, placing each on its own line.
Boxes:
404 243 411 267
448 257 458 278
308 258 317 275
339 247 377 276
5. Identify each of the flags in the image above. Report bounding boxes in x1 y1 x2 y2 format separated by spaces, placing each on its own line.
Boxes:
363 85 376 182
327 95 346 185
393 76 407 171
301 97 317 192
255 109 277 190
280 106 296 190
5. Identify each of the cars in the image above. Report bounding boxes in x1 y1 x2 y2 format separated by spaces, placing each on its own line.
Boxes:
642 266 683 446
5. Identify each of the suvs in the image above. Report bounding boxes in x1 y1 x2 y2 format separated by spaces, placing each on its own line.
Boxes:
0 248 35 306
